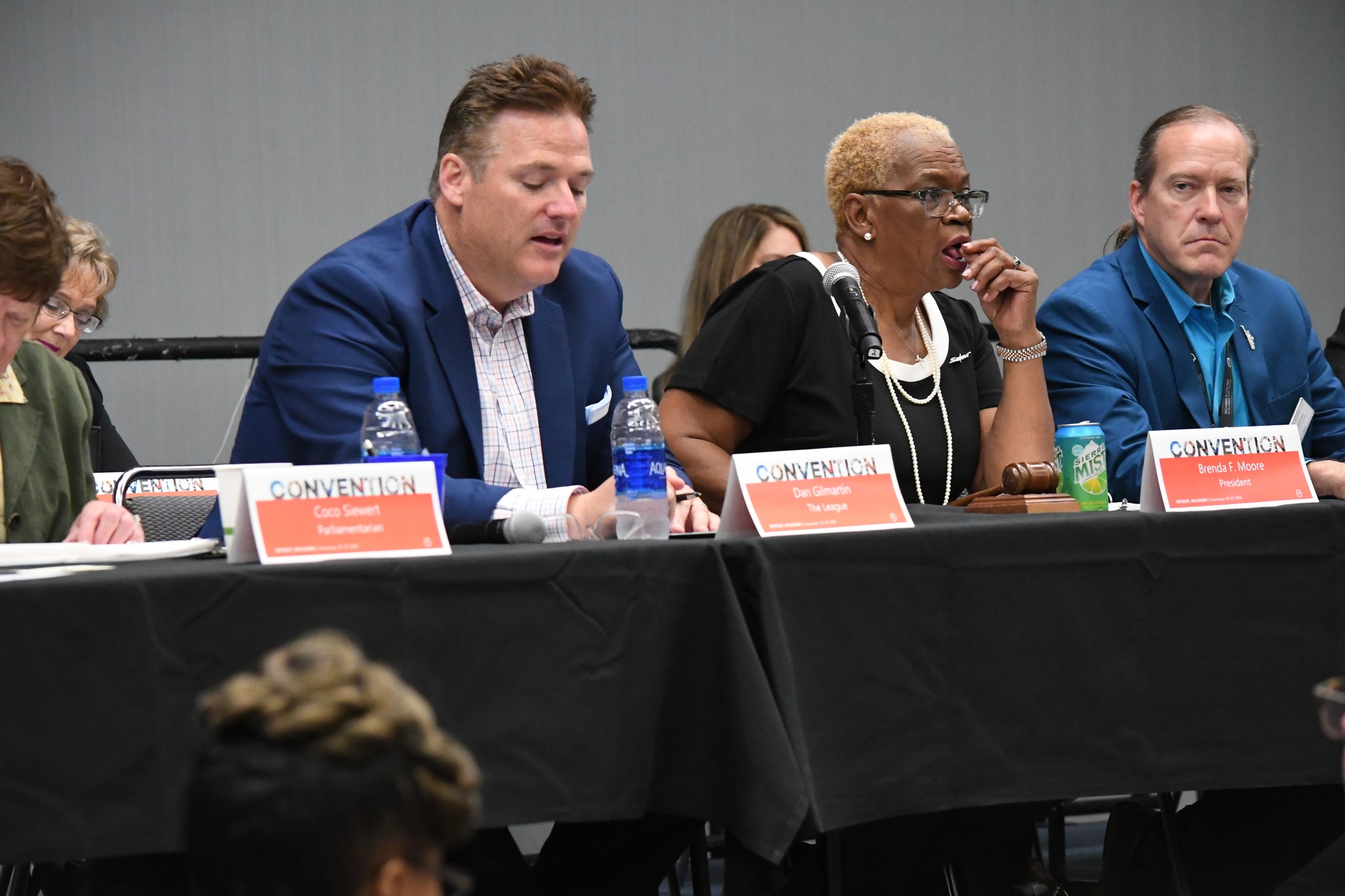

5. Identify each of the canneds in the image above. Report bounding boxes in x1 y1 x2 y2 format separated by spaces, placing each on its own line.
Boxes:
1054 420 1108 511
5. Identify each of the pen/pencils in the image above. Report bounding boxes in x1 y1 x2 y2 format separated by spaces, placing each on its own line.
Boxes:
675 492 701 502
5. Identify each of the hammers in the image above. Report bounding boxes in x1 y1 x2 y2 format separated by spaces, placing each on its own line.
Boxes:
948 461 1059 506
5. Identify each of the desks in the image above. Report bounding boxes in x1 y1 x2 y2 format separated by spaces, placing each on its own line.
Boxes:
0 531 805 896
670 488 1345 896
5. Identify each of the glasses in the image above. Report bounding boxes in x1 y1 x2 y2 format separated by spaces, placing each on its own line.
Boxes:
858 188 990 220
40 296 102 334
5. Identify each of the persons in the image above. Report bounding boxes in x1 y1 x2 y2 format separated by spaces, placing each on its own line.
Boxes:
197 55 724 541
652 205 810 414
27 216 146 474
658 110 1058 516
184 629 485 896
1031 103 1345 498
0 155 148 545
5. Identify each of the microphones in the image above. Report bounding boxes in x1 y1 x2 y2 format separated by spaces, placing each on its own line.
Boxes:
446 512 547 545
822 262 882 360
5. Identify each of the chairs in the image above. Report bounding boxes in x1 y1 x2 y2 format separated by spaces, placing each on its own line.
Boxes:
116 464 221 541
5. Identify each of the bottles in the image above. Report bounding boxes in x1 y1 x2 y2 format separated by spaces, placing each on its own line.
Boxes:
361 376 419 455
611 376 670 540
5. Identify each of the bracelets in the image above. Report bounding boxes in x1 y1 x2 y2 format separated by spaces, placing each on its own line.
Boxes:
994 331 1049 362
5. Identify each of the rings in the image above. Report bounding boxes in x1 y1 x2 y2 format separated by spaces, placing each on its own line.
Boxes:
1012 255 1022 270
130 513 142 529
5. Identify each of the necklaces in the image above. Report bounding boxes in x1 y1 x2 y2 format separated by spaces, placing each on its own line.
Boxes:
839 245 954 509
